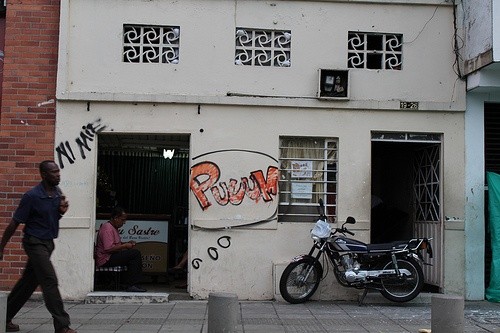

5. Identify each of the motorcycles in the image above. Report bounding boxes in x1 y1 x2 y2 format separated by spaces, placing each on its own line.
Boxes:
279 198 434 307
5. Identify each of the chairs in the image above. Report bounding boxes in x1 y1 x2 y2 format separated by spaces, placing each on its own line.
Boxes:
94 242 126 291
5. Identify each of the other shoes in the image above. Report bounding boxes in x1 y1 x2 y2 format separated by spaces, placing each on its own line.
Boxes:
6 321 20 331
55 326 77 333
127 286 146 292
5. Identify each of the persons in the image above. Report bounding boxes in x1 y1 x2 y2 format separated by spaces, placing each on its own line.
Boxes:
0 160 77 333
94 206 147 292
173 252 188 289
